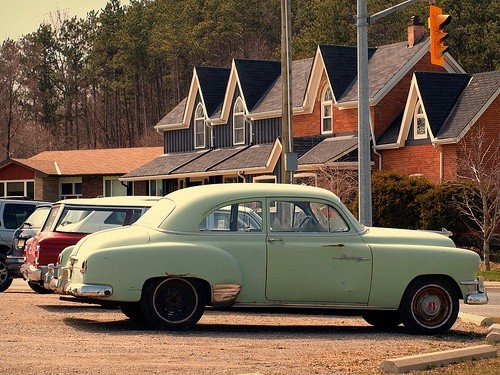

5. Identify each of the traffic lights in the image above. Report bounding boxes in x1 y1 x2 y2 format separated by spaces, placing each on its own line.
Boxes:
430 4 452 68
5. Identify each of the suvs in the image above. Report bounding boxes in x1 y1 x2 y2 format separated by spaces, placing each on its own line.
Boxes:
0 199 49 293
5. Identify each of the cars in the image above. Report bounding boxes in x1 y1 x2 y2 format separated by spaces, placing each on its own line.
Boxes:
4 202 53 280
19 196 165 295
43 182 488 336
